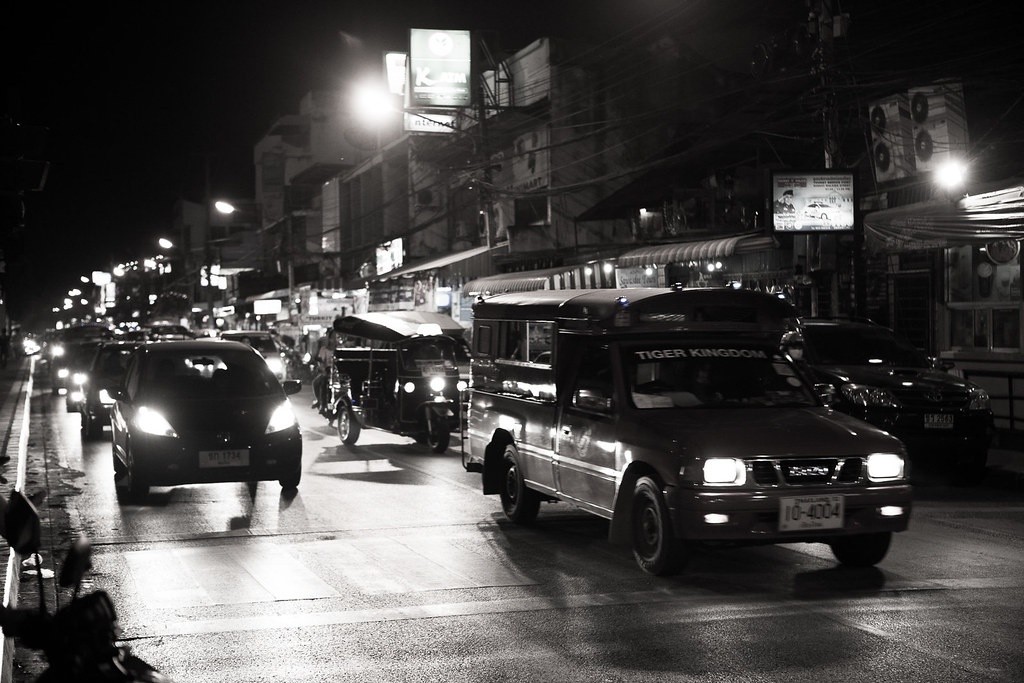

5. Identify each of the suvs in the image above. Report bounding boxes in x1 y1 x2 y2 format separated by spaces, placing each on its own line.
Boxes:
470 287 912 580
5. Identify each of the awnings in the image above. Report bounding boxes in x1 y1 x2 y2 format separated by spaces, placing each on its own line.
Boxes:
464 264 600 293
615 234 767 267
866 179 1024 253
347 241 509 286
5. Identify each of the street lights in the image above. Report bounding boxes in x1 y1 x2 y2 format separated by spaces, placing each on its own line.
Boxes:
158 236 193 321
215 199 295 329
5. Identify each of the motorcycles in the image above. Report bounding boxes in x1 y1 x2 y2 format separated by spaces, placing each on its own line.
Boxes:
329 311 471 453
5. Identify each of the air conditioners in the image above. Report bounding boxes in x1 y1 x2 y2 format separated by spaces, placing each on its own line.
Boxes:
866 93 917 183
477 200 515 236
907 83 969 173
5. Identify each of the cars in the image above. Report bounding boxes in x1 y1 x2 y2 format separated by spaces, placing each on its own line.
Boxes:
779 314 995 450
109 339 303 503
41 325 288 442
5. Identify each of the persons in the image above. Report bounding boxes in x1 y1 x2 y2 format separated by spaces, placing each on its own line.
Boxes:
689 357 724 400
241 337 252 346
0 327 23 370
